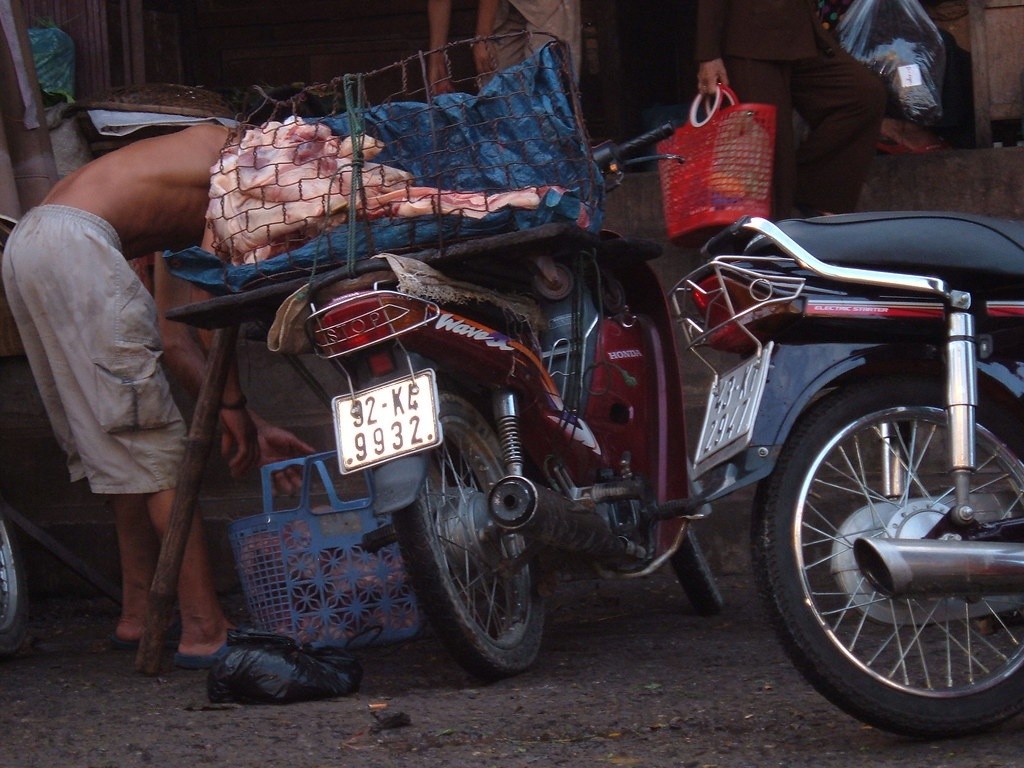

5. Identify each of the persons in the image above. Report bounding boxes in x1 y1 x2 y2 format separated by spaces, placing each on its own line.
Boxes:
696 0 889 216
426 0 583 96
0 87 333 668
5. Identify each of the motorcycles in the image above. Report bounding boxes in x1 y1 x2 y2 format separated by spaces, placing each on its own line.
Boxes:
306 127 724 679
667 208 1024 741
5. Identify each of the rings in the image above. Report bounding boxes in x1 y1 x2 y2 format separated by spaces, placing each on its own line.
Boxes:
703 83 708 87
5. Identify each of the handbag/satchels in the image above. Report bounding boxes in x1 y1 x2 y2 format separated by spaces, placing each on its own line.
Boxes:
229 450 423 649
656 82 778 242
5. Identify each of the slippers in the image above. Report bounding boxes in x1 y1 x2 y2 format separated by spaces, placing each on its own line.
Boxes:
176 629 231 670
110 618 171 649
